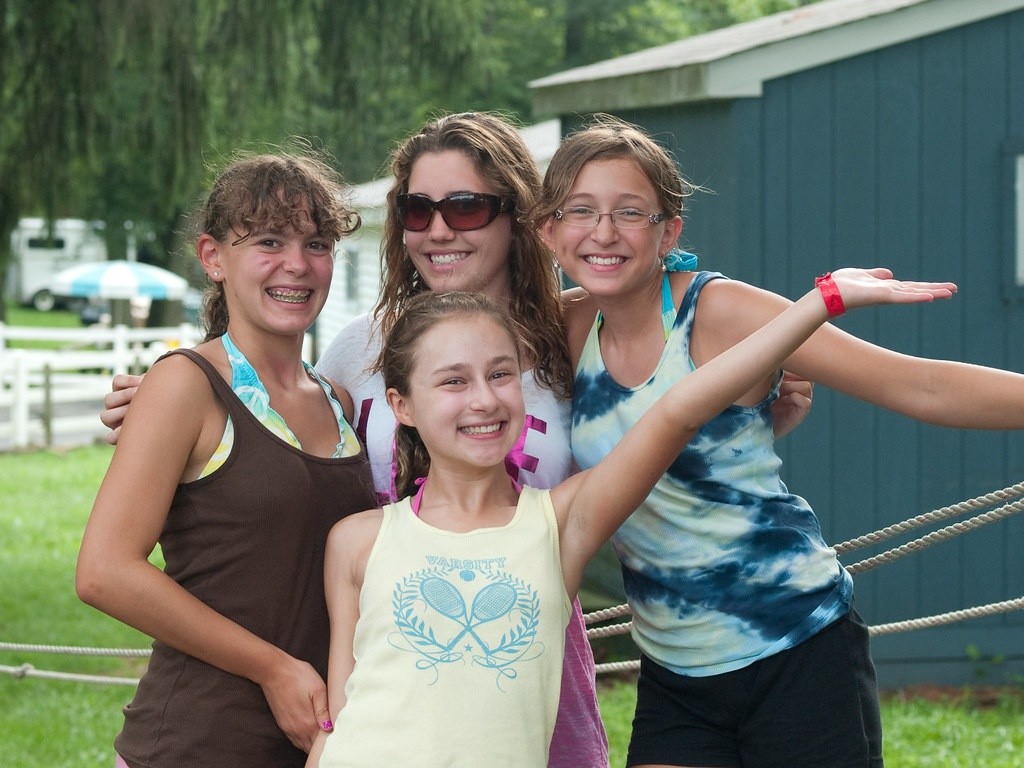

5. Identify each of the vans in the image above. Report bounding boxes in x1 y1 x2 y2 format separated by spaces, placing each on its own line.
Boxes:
11 218 141 312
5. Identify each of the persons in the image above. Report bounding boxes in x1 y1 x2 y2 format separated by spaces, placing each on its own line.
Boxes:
532 122 1023 767
301 267 958 768
101 110 816 768
75 154 378 768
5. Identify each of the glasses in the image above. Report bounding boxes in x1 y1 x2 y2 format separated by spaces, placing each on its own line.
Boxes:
396 193 515 231
554 207 666 229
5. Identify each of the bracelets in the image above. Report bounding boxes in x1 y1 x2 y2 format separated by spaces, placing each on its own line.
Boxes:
815 271 845 318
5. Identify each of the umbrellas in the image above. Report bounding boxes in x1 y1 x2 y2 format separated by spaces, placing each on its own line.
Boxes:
49 259 190 325
